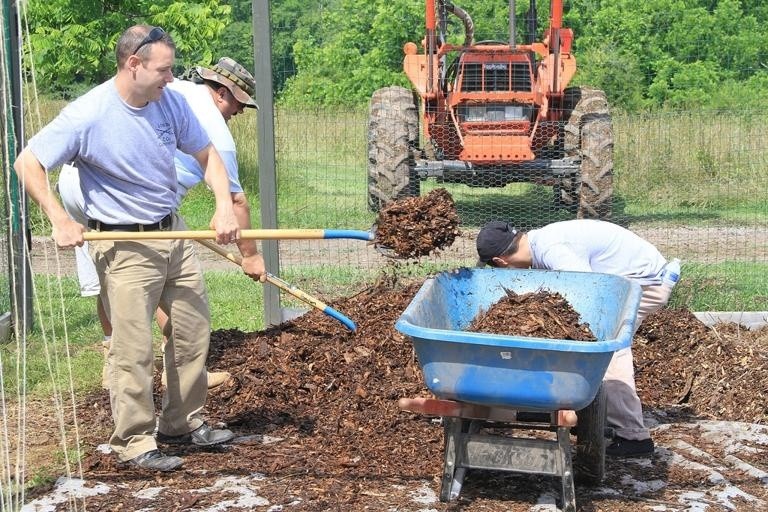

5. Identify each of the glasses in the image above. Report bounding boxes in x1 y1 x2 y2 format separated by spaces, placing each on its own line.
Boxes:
132 27 165 55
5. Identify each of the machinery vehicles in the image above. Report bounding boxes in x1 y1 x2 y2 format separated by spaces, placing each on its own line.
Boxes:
366 0 615 222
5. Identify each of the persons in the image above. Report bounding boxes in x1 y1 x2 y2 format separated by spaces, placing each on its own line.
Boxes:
476 220 673 457
13 26 241 471
57 58 267 388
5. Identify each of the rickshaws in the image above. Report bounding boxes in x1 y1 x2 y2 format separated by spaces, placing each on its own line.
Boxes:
394 266 644 512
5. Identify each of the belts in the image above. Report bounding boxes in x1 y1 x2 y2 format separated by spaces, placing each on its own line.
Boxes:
88 215 171 232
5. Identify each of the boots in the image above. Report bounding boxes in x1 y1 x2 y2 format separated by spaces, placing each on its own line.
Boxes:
161 345 231 389
102 338 112 390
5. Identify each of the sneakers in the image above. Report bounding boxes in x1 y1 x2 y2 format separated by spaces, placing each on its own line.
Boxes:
606 437 654 460
129 424 234 471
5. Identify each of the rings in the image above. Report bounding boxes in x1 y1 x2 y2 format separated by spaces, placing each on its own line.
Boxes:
230 238 236 243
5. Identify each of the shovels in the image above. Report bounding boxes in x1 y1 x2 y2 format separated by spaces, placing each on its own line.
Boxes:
84 189 453 257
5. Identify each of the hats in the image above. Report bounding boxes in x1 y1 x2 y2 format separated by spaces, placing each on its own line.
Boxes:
476 221 520 267
196 56 259 111
